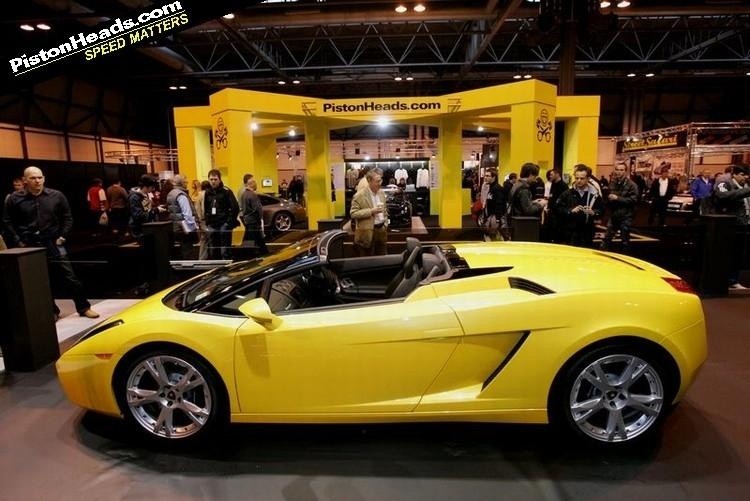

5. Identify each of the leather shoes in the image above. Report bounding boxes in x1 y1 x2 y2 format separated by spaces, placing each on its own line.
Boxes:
79 308 100 318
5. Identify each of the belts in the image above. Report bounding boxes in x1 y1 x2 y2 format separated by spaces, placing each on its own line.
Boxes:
374 222 384 228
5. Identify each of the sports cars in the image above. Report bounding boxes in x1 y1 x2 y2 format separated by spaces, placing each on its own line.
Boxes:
54 230 708 451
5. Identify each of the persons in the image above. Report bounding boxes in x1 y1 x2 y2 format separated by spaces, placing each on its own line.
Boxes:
0 160 748 300
4 166 98 321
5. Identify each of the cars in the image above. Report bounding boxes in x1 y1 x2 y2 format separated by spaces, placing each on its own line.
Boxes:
378 186 413 230
667 193 695 211
254 192 306 234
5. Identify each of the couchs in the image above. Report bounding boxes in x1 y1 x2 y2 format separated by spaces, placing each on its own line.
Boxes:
511 216 662 262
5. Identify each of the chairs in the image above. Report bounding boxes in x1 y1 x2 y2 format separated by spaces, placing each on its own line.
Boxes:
339 238 424 289
334 273 423 303
